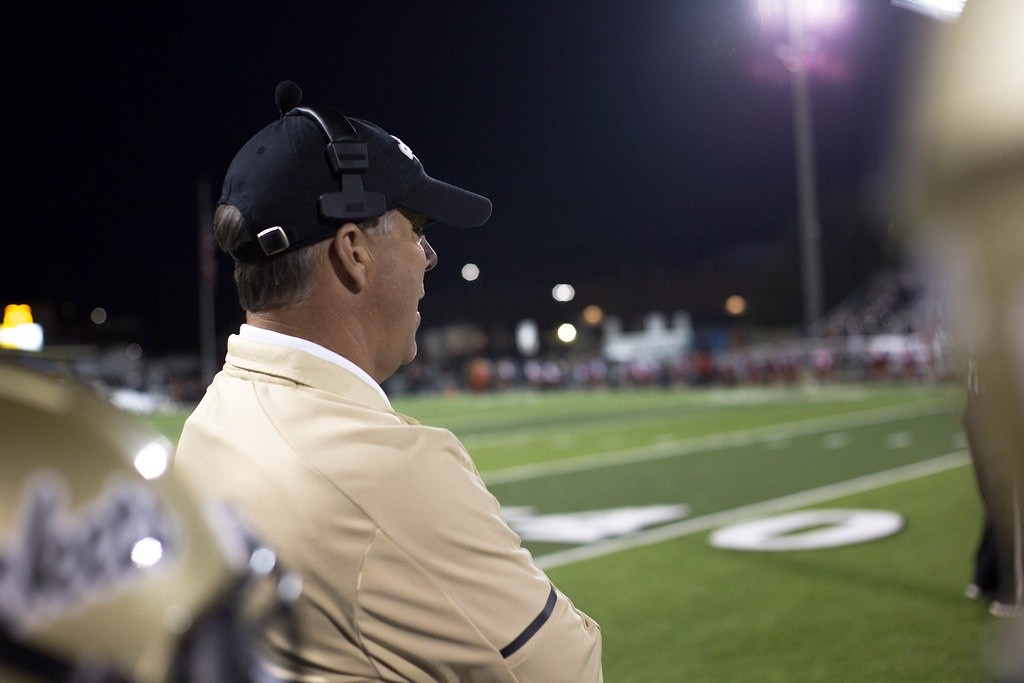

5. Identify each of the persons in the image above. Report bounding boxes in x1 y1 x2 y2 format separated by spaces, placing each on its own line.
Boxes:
174 106 602 683
961 349 1024 616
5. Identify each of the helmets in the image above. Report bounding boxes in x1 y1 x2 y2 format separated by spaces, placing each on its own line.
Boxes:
1 347 302 683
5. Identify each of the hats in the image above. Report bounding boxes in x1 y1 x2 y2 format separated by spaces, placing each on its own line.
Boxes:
207 105 493 258
